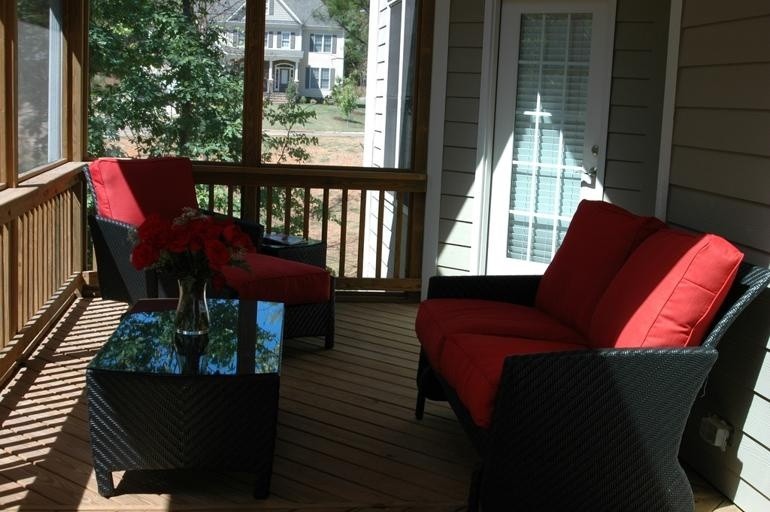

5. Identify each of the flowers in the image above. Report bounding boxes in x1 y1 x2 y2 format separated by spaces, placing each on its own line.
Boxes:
128 207 253 330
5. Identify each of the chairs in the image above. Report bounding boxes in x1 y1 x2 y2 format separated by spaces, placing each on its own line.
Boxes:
84 154 336 353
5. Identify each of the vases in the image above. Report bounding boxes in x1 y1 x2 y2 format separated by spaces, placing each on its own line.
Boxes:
174 277 211 335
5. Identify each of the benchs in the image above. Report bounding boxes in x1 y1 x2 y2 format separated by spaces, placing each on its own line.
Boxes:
413 200 769 512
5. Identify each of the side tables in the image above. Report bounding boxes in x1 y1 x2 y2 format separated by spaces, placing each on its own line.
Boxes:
260 233 325 271
